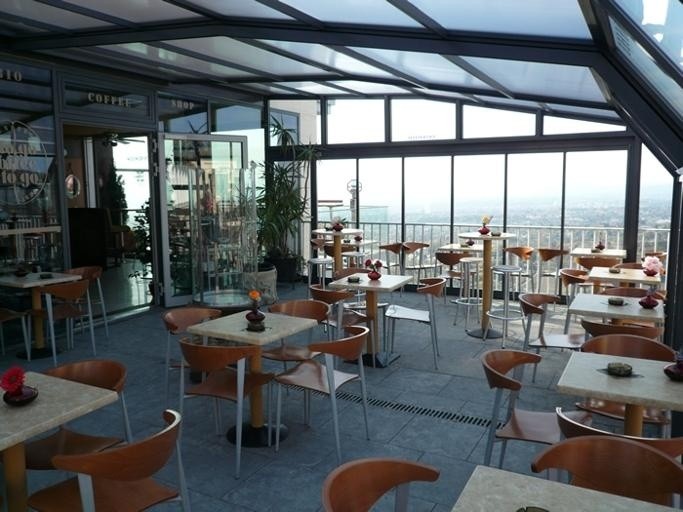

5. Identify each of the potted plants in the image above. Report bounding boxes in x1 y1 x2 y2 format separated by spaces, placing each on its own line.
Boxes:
251 115 324 285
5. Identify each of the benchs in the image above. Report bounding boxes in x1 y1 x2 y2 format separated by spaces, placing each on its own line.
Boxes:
68 208 113 272
111 209 124 248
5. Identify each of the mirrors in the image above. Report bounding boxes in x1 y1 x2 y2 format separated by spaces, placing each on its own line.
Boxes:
65 175 81 200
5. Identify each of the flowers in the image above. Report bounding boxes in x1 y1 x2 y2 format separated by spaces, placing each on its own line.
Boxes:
482 216 493 227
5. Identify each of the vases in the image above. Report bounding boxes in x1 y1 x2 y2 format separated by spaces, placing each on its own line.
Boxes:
478 226 490 235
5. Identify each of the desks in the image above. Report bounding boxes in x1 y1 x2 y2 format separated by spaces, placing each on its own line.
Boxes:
0 227 683 512
